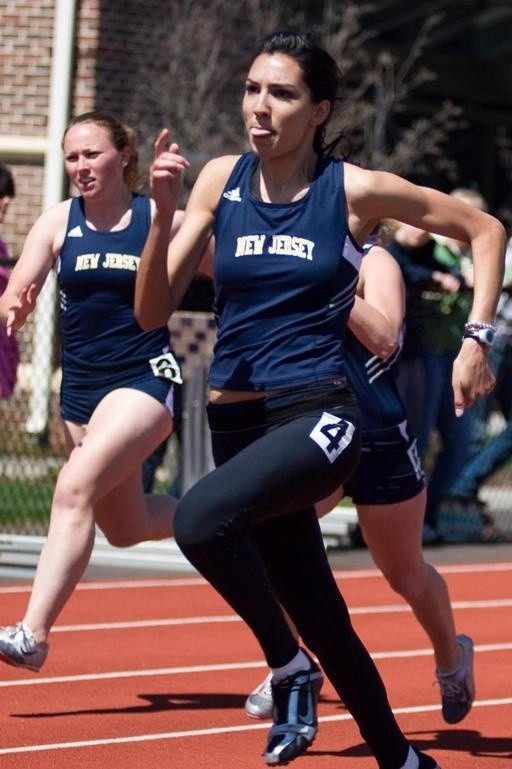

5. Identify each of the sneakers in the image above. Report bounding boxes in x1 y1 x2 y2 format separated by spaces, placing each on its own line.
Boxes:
1 621 49 672
262 647 323 766
412 745 440 769
244 671 275 719
435 634 475 723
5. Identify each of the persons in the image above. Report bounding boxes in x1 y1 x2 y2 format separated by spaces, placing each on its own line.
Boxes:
0 112 217 671
133 36 511 768
